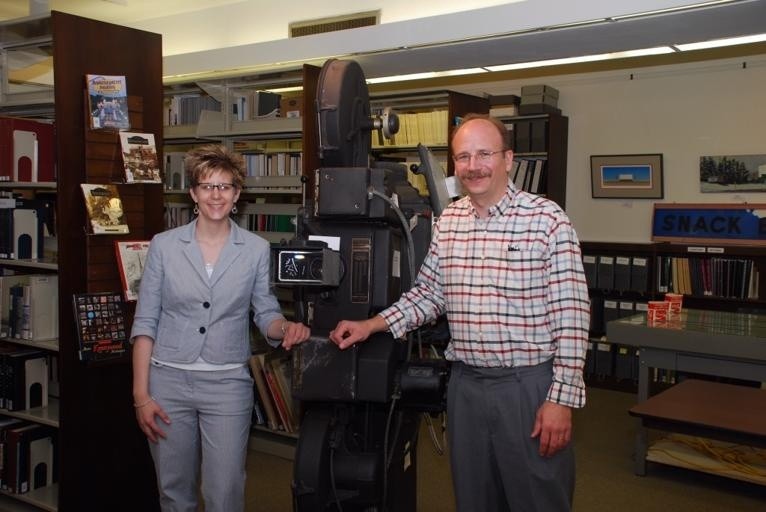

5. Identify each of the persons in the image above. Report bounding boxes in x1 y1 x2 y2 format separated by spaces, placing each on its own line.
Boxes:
327 113 594 512
127 144 313 511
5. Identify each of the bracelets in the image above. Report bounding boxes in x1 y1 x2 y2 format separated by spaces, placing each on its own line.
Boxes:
133 396 155 410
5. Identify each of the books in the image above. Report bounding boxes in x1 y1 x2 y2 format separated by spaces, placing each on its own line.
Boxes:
245 306 299 432
243 153 302 204
72 293 128 362
0 188 51 495
164 152 197 228
237 213 296 280
79 182 131 235
173 95 222 127
113 240 151 301
236 92 282 122
659 254 766 298
370 109 449 195
118 129 163 184
85 73 131 131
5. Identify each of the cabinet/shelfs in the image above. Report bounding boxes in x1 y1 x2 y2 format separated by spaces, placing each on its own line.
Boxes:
368 88 569 218
579 239 765 398
0 10 323 511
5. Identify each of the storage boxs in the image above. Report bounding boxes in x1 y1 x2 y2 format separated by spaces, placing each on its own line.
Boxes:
519 85 560 100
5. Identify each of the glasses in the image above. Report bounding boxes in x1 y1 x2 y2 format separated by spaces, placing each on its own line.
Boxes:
194 182 236 194
453 148 508 163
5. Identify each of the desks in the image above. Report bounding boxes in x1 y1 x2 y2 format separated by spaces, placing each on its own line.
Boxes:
628 379 764 485
607 308 765 405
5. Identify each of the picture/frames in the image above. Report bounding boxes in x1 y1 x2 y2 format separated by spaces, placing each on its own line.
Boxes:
700 155 764 193
589 153 664 199
